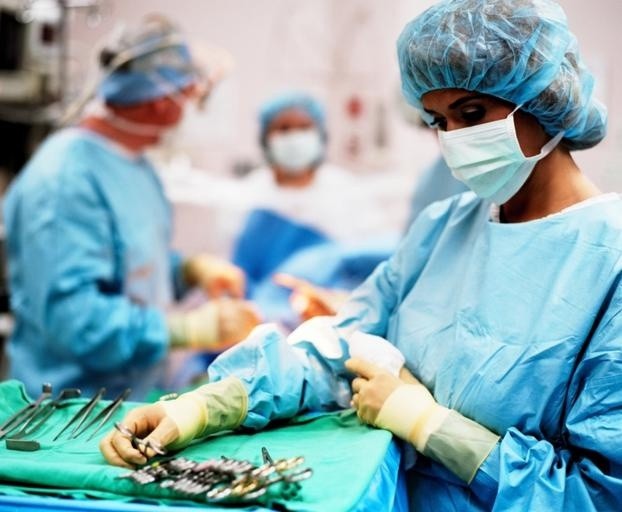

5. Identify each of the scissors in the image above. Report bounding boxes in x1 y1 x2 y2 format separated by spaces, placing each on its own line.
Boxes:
114 422 312 503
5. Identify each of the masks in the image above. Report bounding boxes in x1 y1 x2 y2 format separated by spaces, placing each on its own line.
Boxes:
263 125 322 169
146 92 200 153
437 104 566 207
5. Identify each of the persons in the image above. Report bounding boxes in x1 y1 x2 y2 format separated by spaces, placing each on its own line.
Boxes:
98 0 622 511
0 9 271 401
207 87 387 356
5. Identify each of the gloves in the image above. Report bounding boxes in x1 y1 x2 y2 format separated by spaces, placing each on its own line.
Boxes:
166 251 262 351
99 375 248 471
344 358 502 485
